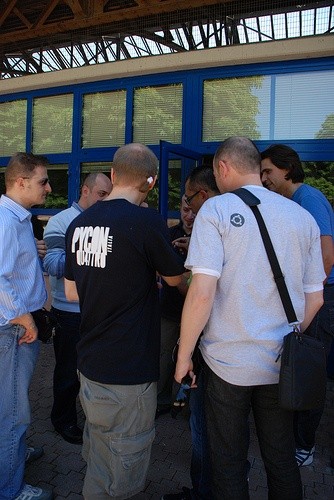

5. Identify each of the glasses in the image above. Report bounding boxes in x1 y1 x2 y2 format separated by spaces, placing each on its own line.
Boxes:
182 190 207 207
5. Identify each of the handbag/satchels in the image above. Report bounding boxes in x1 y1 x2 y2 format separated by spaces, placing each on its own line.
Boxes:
32 308 55 345
275 331 328 415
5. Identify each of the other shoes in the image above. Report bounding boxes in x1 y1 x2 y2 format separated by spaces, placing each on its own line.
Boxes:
59 425 83 445
16 484 54 499
295 445 314 465
25 447 43 465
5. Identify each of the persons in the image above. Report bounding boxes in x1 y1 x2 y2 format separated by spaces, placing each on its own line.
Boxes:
0 151 53 500
45 135 334 500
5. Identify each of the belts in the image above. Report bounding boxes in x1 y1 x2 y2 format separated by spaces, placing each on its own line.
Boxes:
50 309 81 319
